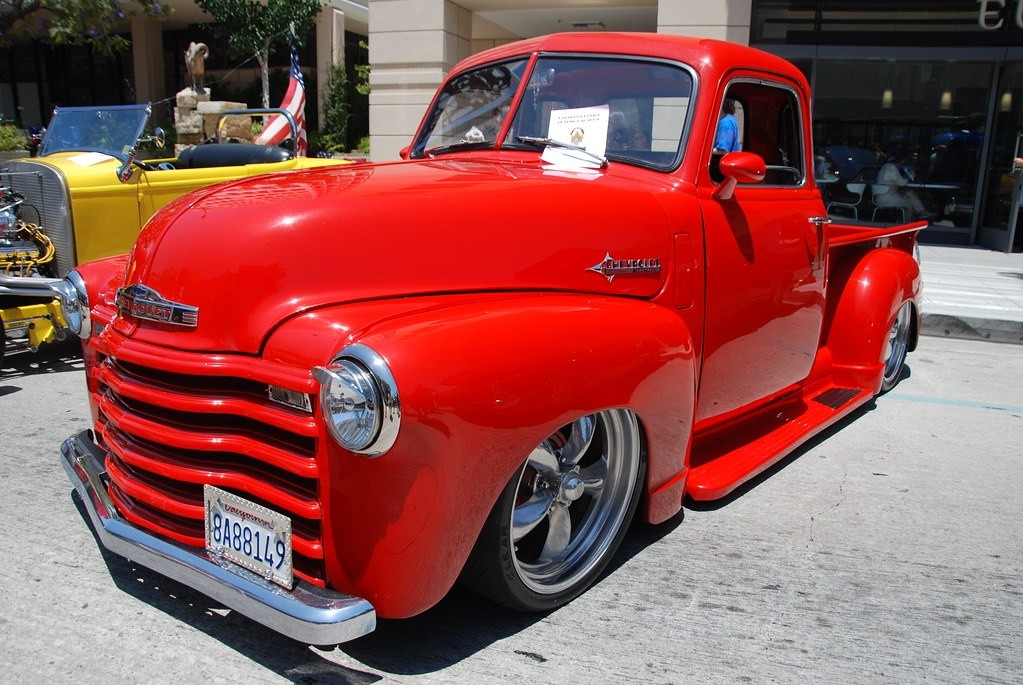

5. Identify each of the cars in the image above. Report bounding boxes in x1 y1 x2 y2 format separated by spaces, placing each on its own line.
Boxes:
0 101 369 358
54 31 929 648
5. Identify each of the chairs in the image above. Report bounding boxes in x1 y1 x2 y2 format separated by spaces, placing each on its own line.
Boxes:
826 183 866 220
867 185 906 226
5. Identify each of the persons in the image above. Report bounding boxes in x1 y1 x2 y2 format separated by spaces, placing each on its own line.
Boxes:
815 135 981 221
712 97 740 154
611 127 650 150
1013 158 1023 167
481 98 514 144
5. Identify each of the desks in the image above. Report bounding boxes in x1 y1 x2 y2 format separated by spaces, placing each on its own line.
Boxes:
908 184 961 189
816 180 838 183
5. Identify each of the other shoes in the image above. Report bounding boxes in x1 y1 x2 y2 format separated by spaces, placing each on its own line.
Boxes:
919 210 938 219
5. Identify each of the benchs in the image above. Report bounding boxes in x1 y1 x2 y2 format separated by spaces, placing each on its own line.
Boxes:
615 148 800 183
178 143 290 170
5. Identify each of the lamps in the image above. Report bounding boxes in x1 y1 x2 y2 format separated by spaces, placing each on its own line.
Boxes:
880 84 894 110
999 91 1014 112
939 89 951 111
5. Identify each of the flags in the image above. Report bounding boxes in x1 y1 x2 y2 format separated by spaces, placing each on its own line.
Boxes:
253 24 308 158
